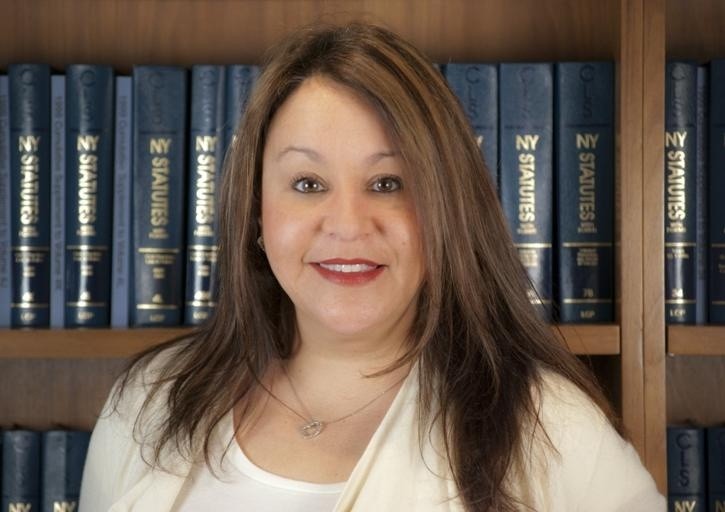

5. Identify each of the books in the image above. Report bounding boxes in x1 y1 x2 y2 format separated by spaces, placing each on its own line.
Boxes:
665 58 725 326
0 430 92 512
667 423 725 512
443 62 616 323
0 62 265 329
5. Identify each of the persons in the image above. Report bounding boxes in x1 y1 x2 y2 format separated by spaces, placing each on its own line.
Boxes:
75 20 668 512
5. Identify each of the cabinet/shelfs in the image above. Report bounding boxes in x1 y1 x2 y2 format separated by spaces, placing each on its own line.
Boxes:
0 0 725 512
